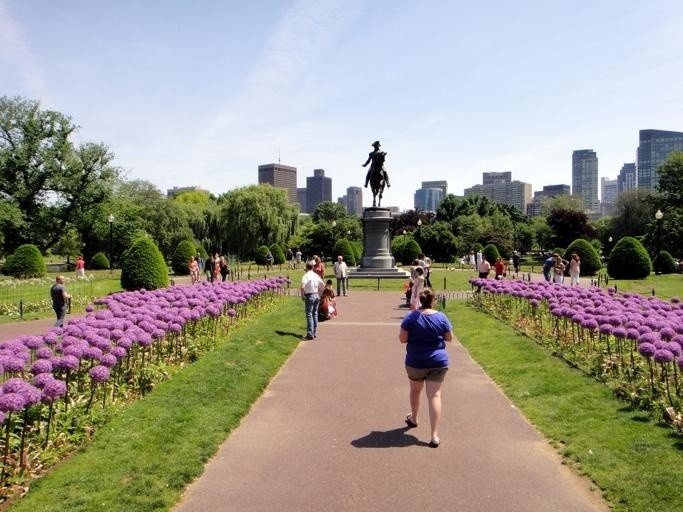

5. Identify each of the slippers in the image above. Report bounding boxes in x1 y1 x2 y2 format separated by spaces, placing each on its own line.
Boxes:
407 413 417 426
431 437 440 447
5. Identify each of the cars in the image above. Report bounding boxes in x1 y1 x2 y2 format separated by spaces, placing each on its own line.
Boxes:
528 250 548 257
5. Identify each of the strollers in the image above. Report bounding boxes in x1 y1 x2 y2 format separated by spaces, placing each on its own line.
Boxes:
403 281 412 304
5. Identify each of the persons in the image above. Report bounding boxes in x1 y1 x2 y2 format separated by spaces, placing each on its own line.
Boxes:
399 287 453 448
541 253 581 287
362 140 391 187
404 253 433 310
286 248 337 339
188 252 229 285
266 252 274 272
75 256 85 278
50 275 70 328
333 255 349 297
468 250 520 292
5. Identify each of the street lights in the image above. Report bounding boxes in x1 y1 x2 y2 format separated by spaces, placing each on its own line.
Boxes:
416 218 422 246
607 236 613 251
106 213 115 271
654 208 663 252
402 229 406 242
347 229 351 239
330 221 336 247
